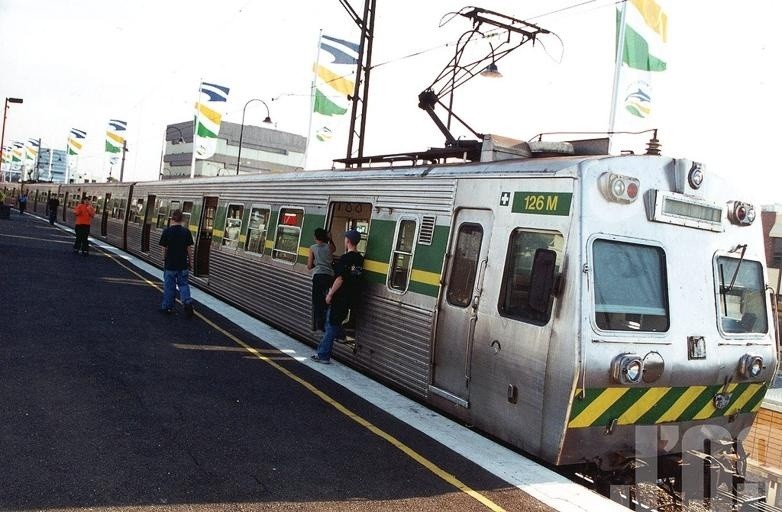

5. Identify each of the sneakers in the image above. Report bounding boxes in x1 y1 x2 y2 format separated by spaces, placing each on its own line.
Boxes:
72 252 90 257
158 302 195 319
336 336 346 342
311 354 329 364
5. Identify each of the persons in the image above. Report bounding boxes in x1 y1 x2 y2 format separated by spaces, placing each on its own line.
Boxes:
0 187 28 216
70 197 95 255
47 193 59 227
157 209 194 316
307 227 365 364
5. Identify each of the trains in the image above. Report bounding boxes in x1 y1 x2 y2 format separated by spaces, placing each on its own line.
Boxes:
2 154 779 468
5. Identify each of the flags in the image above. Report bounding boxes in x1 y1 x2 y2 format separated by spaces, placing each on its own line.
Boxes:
624 87 651 122
0 83 227 168
309 28 364 142
618 0 671 72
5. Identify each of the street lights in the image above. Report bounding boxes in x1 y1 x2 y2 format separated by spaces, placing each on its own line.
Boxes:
0 97 24 172
229 98 272 175
158 124 185 183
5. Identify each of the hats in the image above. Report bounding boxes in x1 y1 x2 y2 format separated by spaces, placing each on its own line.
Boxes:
344 230 361 245
